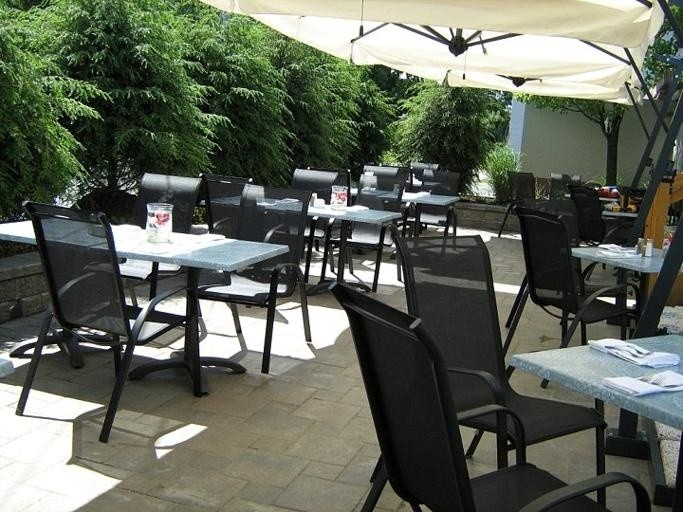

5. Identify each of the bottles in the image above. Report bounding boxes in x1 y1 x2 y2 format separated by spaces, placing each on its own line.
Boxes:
636 238 644 256
644 238 653 257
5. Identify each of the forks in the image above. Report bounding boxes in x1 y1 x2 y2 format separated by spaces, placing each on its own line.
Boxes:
604 344 653 360
637 373 653 382
650 375 666 387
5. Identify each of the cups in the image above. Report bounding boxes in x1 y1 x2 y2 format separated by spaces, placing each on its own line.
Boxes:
313 198 325 209
